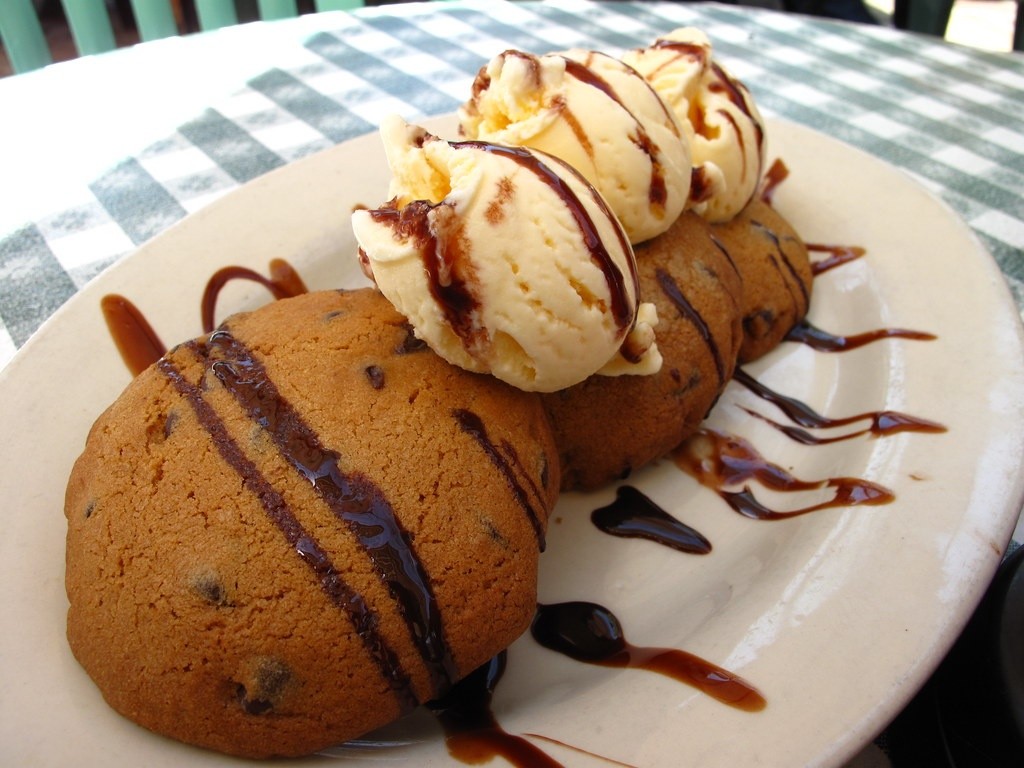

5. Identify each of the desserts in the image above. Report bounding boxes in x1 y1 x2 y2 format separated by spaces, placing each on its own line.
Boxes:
61 23 814 753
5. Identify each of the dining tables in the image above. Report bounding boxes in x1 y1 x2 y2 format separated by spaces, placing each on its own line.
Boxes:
0 1 1024 768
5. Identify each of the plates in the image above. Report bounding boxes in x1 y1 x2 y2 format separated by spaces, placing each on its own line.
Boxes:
2 114 1024 767
